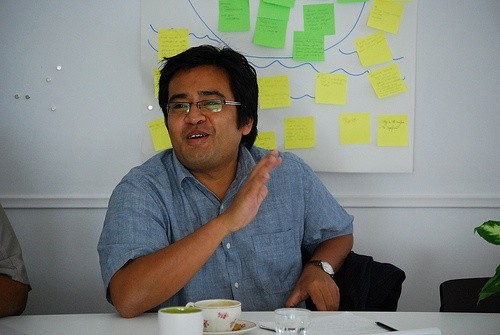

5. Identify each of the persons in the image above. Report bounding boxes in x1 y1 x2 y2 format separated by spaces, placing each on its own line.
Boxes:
97 44 354 318
0 203 33 319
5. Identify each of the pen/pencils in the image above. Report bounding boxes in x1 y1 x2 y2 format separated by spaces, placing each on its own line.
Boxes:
375 321 399 332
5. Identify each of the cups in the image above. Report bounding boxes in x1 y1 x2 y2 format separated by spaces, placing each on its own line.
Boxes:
274 308 312 335
158 307 203 335
187 299 242 331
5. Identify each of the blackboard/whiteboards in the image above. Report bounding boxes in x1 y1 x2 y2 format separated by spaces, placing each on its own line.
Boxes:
1 0 500 209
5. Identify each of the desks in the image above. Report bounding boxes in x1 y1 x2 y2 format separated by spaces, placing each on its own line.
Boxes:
1 311 500 335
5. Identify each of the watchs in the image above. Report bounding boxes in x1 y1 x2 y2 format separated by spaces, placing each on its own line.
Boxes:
306 260 335 278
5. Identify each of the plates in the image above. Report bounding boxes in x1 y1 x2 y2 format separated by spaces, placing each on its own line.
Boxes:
203 320 256 335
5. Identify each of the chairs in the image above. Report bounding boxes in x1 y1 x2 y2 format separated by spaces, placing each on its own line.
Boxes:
301 245 407 312
439 276 491 312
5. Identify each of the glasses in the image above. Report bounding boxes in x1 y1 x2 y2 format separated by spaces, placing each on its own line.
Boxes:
166 98 241 112
